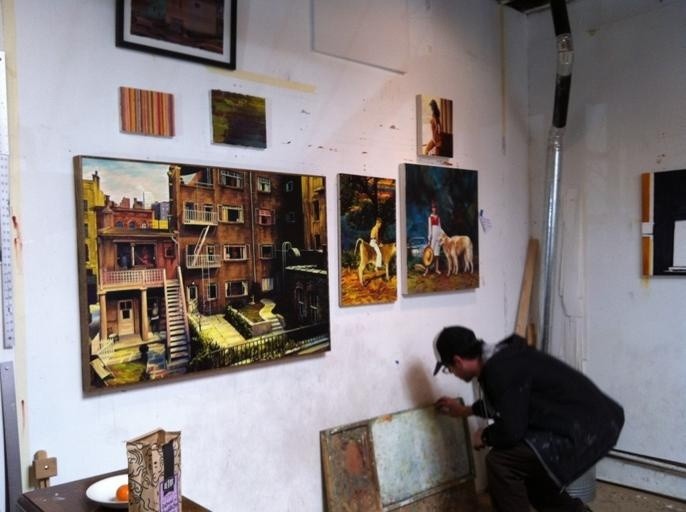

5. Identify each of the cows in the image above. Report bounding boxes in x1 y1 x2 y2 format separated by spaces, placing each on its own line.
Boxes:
354 237 396 287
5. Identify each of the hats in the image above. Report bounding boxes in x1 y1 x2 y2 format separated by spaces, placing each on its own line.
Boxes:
433 325 478 378
421 246 434 267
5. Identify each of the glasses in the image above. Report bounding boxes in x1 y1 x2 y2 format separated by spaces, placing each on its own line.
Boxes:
441 359 454 376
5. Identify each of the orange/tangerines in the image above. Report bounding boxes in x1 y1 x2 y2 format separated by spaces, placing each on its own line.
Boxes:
117 484 128 501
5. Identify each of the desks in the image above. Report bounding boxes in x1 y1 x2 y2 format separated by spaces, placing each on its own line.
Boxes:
15 467 211 512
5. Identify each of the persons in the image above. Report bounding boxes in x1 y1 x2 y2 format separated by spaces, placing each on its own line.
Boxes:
369 217 383 274
423 202 444 277
433 325 625 512
424 99 442 156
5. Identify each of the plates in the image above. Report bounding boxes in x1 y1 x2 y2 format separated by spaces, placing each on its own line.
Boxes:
85 474 128 508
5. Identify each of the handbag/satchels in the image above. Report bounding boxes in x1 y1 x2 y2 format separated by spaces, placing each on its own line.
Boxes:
124 429 184 512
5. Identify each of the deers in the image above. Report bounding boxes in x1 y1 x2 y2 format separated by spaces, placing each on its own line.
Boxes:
435 231 474 278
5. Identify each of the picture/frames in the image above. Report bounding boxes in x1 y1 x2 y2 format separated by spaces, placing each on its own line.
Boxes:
114 1 237 71
